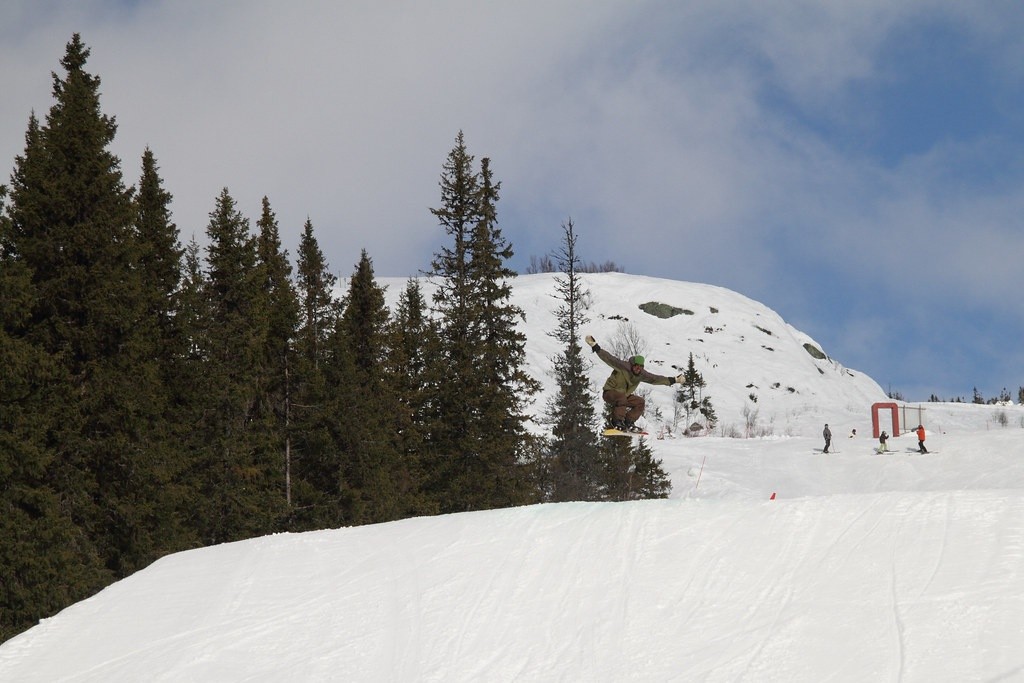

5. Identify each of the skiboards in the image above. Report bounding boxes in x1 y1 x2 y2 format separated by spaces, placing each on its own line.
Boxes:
813 448 841 455
905 450 939 456
871 447 900 455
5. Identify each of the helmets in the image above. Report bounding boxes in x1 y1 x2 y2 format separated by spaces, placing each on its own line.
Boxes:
918 425 923 429
882 431 887 434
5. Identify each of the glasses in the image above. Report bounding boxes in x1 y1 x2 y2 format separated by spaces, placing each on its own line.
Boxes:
635 364 644 368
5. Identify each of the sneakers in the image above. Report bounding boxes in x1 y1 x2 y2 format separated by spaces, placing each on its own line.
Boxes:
624 420 644 433
609 417 628 432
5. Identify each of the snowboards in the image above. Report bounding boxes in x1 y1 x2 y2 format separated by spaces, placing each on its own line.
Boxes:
599 429 649 436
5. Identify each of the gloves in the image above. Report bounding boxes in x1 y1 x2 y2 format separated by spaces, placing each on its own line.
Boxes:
668 374 687 385
585 335 601 353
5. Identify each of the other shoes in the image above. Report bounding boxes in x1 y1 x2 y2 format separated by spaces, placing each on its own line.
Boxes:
823 450 829 453
917 450 923 452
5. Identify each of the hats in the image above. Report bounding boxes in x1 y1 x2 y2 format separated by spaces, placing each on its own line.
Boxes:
635 355 644 365
825 424 828 426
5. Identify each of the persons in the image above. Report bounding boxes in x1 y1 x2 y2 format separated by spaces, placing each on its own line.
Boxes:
585 335 687 431
822 423 833 454
878 431 890 454
917 425 929 453
